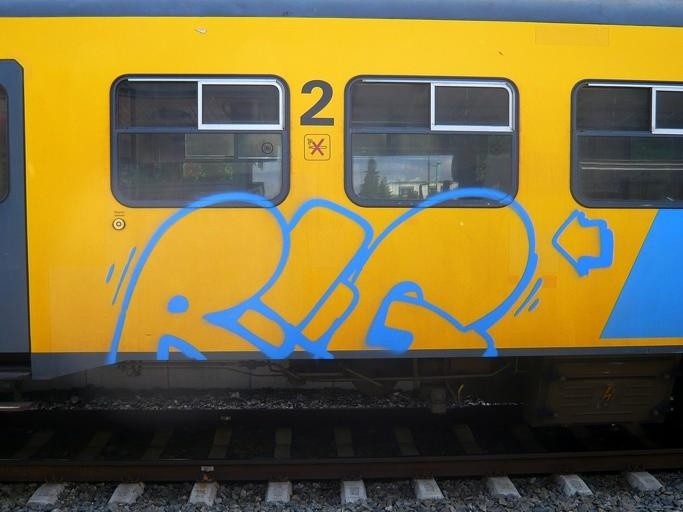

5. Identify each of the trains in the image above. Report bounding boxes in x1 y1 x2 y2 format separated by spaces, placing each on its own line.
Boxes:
0 2 681 430
